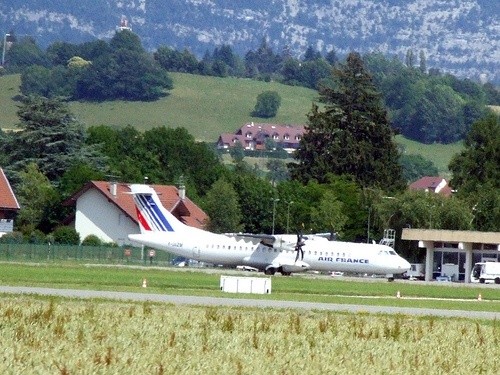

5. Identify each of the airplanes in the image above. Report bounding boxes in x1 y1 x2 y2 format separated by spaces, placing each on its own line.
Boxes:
123 183 413 277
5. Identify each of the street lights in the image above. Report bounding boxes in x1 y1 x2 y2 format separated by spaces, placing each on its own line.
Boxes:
287 201 294 234
272 199 280 235
2 33 11 65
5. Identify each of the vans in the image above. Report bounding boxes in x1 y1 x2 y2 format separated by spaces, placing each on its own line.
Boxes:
478 261 500 284
